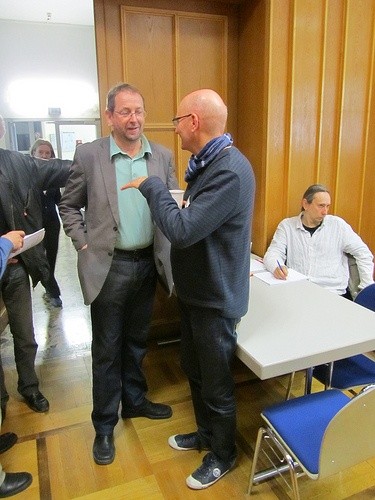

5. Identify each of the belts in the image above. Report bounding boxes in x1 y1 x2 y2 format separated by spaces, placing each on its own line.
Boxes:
114 246 153 258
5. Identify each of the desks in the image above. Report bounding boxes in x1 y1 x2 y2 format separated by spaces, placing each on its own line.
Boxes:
158 253 375 484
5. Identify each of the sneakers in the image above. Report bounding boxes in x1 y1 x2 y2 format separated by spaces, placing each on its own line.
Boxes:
186 451 237 489
169 430 209 450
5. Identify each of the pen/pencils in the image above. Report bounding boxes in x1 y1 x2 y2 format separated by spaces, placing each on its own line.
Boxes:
276 258 288 281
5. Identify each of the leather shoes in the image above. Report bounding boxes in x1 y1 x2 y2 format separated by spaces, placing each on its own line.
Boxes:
122 401 172 420
91 425 116 464
0 431 17 452
0 471 32 496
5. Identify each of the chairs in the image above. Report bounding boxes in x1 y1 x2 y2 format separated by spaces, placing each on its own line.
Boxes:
285 283 375 401
248 383 375 500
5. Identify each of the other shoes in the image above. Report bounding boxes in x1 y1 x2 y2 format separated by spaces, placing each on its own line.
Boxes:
42 293 63 306
25 392 48 413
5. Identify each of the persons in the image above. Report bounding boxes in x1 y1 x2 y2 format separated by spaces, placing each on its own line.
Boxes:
31 139 62 307
1 117 73 420
262 185 375 302
58 84 173 465
0 231 32 498
122 89 256 490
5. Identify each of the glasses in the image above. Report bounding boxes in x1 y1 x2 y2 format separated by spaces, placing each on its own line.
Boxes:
114 108 147 117
171 113 191 126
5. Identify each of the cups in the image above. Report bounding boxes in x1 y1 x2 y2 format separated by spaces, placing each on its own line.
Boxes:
169 190 185 209
